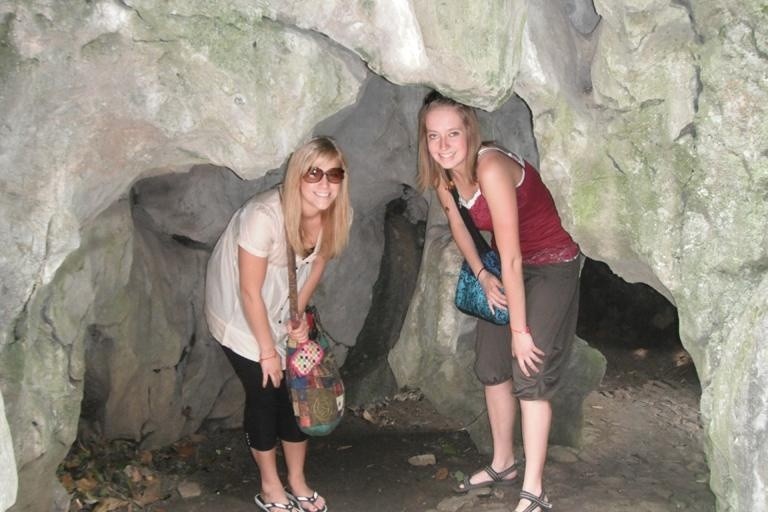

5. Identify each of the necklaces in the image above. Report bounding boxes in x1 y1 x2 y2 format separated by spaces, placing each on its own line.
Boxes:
457 193 464 209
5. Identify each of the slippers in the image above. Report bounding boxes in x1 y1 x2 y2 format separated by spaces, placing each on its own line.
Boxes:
254 494 304 512
284 486 327 512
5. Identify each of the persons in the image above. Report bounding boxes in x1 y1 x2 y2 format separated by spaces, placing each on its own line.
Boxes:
204 135 353 512
418 87 581 512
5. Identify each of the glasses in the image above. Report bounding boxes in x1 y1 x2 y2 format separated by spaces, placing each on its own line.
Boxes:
303 166 344 183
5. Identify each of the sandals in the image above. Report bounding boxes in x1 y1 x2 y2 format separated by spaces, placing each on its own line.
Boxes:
512 489 553 512
453 464 520 493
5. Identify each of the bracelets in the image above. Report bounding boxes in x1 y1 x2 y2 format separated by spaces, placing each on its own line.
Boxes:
260 350 277 361
510 326 531 334
475 266 486 279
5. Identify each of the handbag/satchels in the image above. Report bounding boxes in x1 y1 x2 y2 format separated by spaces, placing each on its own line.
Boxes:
455 251 509 326
287 305 346 436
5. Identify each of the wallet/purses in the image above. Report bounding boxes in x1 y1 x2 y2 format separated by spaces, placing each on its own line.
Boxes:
289 340 324 377
